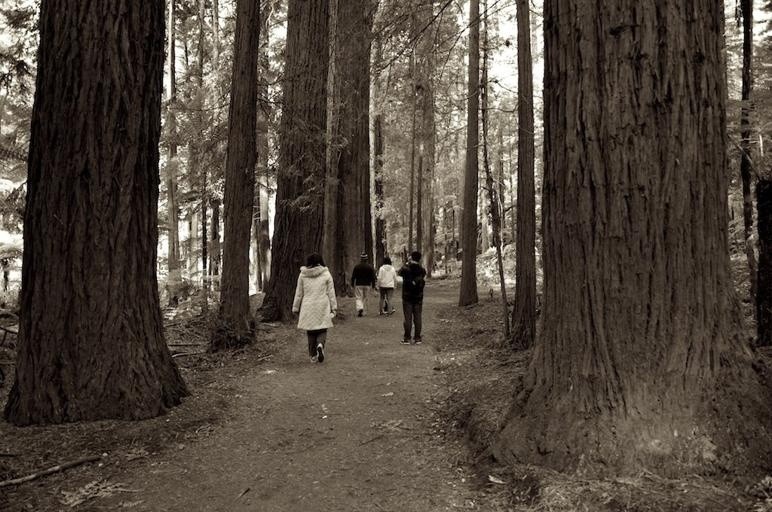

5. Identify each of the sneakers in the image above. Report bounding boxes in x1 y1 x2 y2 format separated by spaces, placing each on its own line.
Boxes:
399 339 422 345
357 307 368 317
309 342 325 363
378 308 396 315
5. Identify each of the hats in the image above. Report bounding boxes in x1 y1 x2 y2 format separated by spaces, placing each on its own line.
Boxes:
360 252 368 260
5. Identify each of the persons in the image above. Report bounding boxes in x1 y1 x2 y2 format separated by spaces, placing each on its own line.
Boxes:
351 252 375 317
376 257 397 315
292 252 337 363
398 252 426 344
